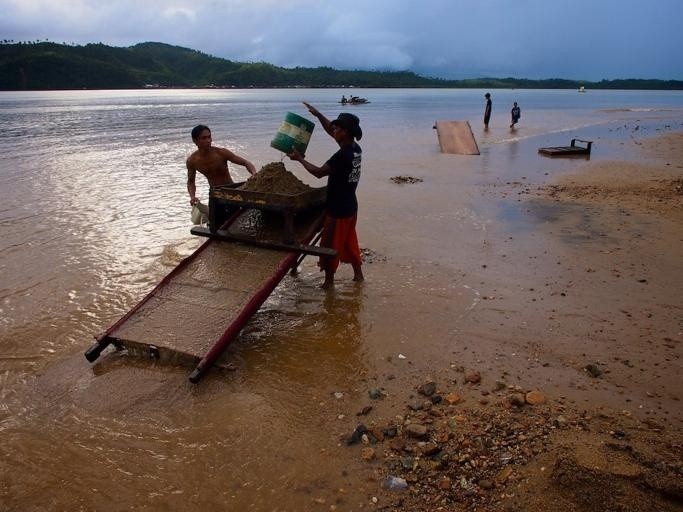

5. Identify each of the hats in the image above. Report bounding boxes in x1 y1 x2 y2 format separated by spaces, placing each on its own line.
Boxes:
331 113 362 141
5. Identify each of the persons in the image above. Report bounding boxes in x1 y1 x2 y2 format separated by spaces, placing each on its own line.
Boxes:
482 92 491 130
287 99 364 289
185 121 257 206
340 94 346 102
508 101 520 129
346 94 352 102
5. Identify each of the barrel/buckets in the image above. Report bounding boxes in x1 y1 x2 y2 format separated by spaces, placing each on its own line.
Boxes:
269 111 314 162
